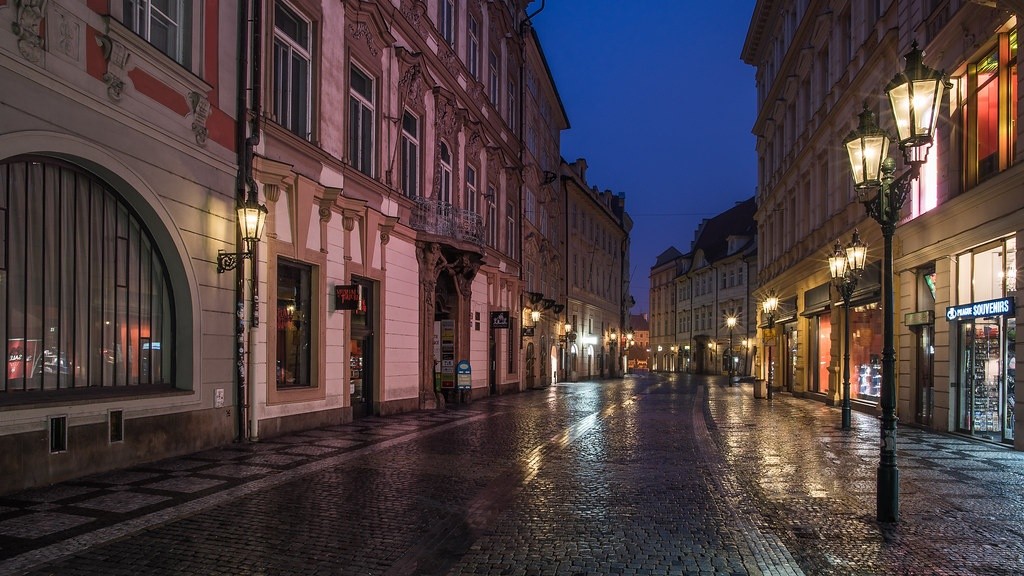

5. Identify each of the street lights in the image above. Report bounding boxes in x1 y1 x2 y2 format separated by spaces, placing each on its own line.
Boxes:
727 317 737 387
762 287 780 399
842 35 950 525
826 227 870 431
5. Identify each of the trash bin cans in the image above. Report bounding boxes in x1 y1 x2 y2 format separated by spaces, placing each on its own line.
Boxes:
754 378 767 399
